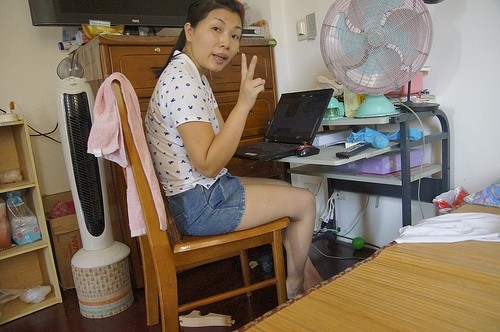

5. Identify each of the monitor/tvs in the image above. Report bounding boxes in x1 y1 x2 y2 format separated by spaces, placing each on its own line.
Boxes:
29 0 192 35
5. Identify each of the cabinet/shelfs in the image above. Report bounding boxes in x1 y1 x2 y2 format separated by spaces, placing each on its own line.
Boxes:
79 33 281 181
283 109 450 243
0 119 62 325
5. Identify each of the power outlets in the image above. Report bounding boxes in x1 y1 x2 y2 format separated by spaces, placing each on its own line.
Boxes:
303 183 314 192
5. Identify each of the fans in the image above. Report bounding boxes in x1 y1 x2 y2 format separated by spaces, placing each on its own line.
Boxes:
320 0 434 119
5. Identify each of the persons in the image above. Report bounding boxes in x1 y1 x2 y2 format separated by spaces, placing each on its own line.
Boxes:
145 0 324 299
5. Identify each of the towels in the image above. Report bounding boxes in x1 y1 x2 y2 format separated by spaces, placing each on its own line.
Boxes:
86 72 168 238
394 212 500 244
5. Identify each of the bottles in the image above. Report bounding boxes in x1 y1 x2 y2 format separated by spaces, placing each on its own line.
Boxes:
327 96 340 120
343 85 362 117
0 200 15 251
261 248 272 272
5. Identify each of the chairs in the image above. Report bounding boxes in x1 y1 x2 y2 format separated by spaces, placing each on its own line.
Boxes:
98 72 291 332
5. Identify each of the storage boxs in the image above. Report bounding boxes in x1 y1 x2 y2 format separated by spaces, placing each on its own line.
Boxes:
46 213 82 291
329 148 423 176
343 66 431 117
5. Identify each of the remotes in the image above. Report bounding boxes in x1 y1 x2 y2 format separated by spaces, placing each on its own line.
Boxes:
336 142 370 158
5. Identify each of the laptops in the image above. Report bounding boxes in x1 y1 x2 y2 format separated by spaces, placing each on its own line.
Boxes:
234 88 334 161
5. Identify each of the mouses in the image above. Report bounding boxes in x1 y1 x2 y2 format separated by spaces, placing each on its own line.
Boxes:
296 145 320 157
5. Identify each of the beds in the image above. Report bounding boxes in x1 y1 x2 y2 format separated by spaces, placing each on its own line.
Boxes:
229 199 500 332
